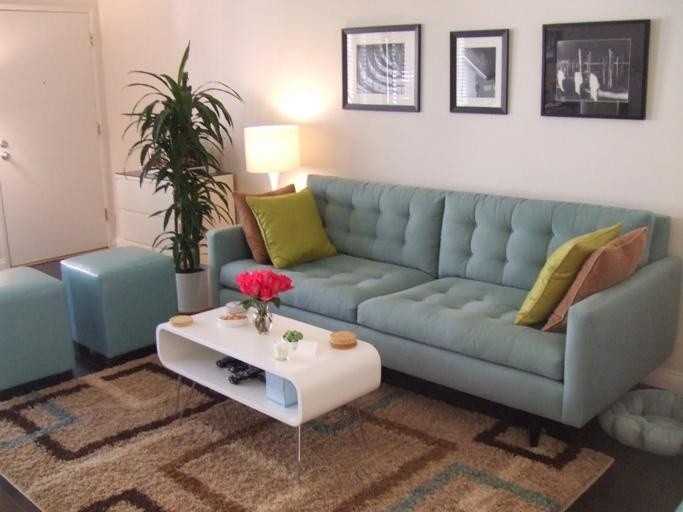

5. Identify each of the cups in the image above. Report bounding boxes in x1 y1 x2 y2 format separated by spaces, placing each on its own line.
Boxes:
272 341 287 361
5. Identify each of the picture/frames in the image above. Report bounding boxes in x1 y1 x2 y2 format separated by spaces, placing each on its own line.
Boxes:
449 29 509 114
342 23 418 112
540 18 651 121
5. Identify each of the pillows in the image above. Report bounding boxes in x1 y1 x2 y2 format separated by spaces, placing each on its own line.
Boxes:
229 183 338 271
513 223 648 337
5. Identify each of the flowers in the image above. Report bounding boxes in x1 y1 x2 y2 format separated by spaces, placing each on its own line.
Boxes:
234 266 294 332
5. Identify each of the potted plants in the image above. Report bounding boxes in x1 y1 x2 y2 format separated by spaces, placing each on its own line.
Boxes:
119 41 246 315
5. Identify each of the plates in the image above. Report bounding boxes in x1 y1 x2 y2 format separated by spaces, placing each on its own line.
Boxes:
216 314 247 326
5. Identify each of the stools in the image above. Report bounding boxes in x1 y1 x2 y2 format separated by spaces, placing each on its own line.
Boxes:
0 246 178 391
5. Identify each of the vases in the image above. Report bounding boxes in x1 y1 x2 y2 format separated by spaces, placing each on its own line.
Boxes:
250 301 272 333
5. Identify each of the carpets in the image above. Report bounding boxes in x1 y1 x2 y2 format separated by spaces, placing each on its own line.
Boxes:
0 341 682 512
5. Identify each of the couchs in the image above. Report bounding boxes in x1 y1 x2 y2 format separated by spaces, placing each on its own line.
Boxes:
207 172 682 445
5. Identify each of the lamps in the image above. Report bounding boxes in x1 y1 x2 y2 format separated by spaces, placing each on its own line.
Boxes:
243 125 301 191
